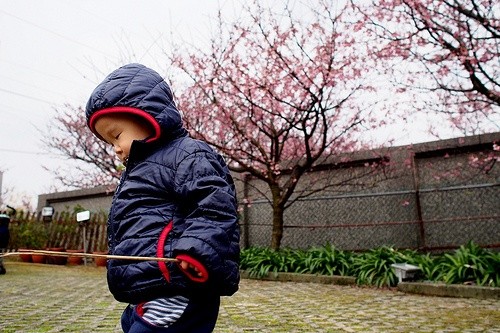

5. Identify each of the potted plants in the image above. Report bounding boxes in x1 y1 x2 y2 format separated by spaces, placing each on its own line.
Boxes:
18 221 84 265
93 232 109 266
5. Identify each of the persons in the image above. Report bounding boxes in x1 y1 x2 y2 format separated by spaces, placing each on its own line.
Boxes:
85 63 244 333
